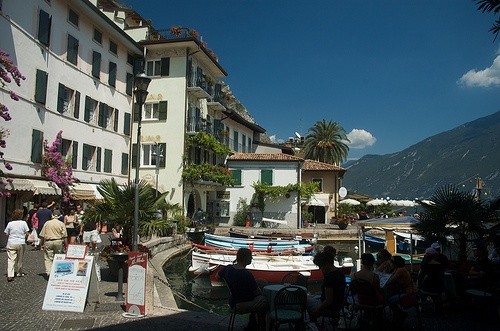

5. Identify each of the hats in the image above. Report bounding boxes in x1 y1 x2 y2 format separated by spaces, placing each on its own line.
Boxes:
52 210 63 219
426 243 440 254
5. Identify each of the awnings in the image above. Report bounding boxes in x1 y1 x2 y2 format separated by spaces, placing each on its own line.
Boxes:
0 177 34 191
30 180 62 195
67 183 104 200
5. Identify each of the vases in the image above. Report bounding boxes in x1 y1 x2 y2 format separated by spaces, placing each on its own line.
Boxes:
106 253 129 283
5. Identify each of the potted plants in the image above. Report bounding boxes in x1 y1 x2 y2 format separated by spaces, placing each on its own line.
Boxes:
338 218 348 230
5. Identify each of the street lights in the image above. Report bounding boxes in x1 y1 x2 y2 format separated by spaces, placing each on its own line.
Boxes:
131 73 153 255
153 152 163 197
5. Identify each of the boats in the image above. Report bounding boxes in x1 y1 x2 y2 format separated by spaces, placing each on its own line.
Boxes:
188 233 455 288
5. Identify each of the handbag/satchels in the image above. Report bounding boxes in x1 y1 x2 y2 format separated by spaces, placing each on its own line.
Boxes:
27 228 38 242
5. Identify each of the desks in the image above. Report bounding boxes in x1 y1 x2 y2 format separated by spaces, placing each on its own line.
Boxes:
437 272 472 307
263 284 308 311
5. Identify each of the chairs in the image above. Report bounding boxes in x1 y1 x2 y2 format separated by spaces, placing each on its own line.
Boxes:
321 274 421 328
280 271 307 287
269 286 308 331
222 278 265 331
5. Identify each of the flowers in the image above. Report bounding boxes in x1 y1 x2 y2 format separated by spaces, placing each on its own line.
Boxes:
43 130 79 203
99 243 152 259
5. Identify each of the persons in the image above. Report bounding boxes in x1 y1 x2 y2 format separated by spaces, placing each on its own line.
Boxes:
217 248 269 331
23 201 124 254
414 212 419 217
39 209 68 280
305 242 500 331
194 208 203 226
4 209 30 281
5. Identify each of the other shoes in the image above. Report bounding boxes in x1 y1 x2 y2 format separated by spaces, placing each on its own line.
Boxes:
17 273 25 277
246 321 269 331
395 313 408 320
7 277 14 282
